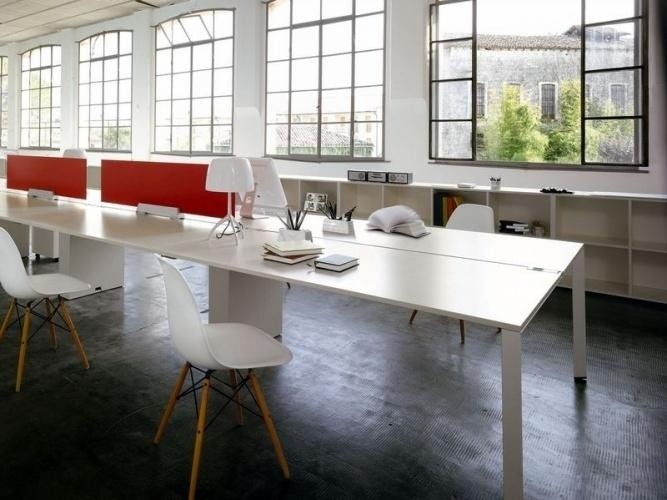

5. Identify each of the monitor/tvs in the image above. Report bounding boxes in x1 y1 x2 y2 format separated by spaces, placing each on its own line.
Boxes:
237 157 288 221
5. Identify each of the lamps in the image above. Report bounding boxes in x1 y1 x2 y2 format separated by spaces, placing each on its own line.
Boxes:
204 157 255 245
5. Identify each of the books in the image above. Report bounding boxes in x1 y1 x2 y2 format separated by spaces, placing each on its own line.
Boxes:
498 220 529 237
314 254 360 273
263 240 325 256
264 251 324 264
457 182 476 189
366 204 431 240
433 191 465 227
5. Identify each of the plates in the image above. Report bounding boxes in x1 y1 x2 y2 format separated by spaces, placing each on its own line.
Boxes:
457 182 477 189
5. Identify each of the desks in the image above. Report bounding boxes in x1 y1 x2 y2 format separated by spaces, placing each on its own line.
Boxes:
1 178 588 500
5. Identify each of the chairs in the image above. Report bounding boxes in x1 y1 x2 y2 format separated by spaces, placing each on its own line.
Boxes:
152 251 293 500
0 227 92 394
408 203 502 344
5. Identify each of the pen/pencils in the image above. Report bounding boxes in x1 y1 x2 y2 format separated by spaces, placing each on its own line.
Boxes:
278 208 309 230
319 201 336 219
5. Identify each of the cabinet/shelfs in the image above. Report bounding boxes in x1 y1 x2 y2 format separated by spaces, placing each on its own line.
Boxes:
278 174 666 306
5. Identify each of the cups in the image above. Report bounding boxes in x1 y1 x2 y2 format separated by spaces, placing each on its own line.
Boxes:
490 181 501 192
